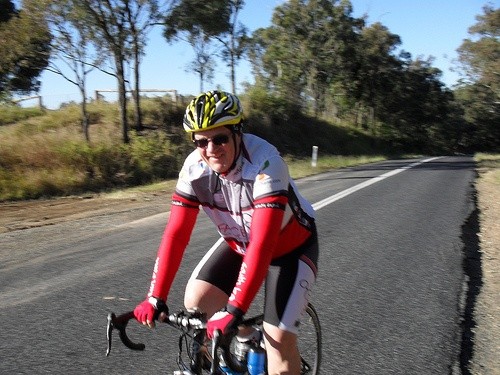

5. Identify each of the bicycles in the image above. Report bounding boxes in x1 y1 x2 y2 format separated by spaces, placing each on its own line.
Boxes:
105 301 322 375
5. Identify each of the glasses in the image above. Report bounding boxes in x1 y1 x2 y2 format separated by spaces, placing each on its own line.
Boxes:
192 131 234 148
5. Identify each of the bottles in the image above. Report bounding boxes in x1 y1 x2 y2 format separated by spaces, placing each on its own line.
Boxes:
246 340 268 375
219 354 248 375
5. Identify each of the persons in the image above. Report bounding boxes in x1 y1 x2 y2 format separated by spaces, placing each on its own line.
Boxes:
133 89 319 375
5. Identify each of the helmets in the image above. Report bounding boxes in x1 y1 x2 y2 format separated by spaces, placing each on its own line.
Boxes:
183 90 244 141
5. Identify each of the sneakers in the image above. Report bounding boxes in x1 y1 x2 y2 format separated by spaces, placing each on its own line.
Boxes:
234 327 263 365
